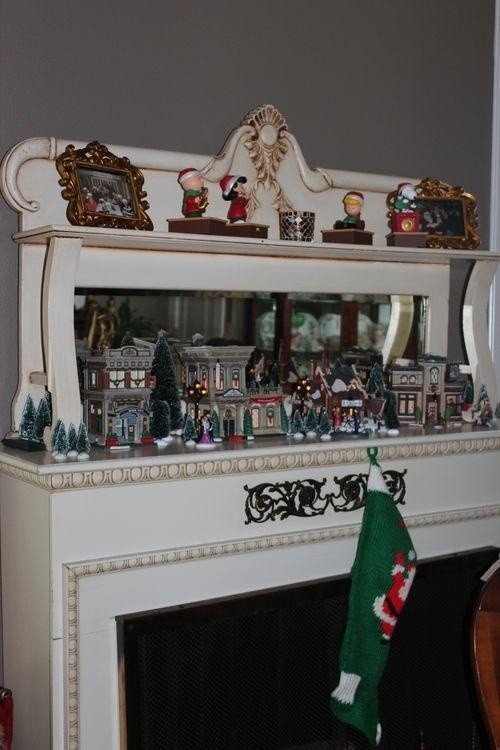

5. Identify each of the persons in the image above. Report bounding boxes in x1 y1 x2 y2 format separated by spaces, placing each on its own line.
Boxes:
220 175 252 224
76 179 138 218
333 192 364 231
177 168 209 217
422 204 458 238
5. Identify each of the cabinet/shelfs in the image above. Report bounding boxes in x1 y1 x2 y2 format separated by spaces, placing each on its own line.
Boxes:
0 138 500 749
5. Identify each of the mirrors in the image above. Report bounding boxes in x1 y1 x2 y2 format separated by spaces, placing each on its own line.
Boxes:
74 296 434 359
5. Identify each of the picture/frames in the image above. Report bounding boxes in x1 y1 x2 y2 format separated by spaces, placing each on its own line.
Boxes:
386 177 481 251
55 140 154 232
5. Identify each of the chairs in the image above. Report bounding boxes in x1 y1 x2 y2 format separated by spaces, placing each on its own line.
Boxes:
471 556 500 749
